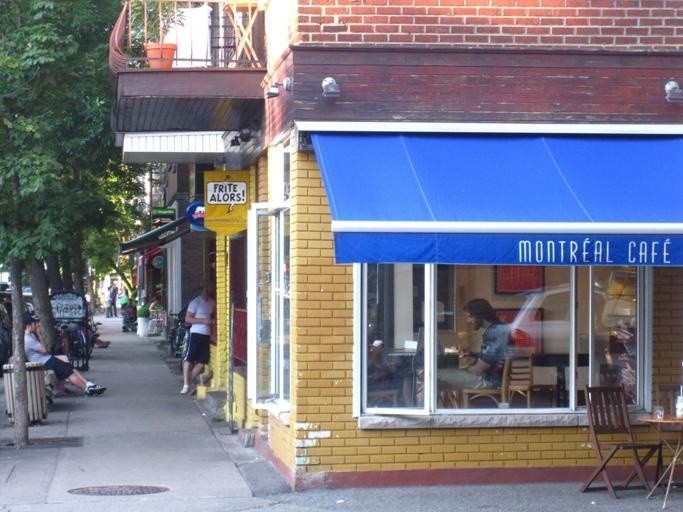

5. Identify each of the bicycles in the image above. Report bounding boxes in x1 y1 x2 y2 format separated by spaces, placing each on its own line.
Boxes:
166 310 190 358
94 300 105 315
51 320 101 372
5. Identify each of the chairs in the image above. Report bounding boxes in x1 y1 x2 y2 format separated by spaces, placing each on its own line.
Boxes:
366 339 539 406
580 380 668 500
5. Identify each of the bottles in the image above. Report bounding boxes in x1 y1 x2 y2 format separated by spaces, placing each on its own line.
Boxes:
676 397 683 419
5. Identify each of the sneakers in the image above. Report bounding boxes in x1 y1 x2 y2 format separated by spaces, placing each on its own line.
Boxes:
84 384 106 396
180 384 190 394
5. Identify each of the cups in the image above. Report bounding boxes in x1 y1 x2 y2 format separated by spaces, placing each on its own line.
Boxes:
498 403 509 409
652 405 664 420
195 383 206 400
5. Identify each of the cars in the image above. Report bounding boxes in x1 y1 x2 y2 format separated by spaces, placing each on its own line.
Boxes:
0 281 34 375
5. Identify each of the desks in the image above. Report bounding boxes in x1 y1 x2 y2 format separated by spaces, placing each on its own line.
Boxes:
529 351 592 407
636 412 683 512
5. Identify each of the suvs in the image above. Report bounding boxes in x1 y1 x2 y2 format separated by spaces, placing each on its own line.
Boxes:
479 284 610 402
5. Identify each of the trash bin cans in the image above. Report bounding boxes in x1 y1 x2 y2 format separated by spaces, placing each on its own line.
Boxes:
2 360 48 426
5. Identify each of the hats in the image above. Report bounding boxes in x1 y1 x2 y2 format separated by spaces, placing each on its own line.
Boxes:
24 315 39 327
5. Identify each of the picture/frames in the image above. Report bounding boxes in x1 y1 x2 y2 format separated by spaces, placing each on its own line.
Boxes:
492 308 545 359
491 263 546 294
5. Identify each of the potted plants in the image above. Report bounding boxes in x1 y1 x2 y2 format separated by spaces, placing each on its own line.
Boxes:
136 307 150 337
121 0 178 71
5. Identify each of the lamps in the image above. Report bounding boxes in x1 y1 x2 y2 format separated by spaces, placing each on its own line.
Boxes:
321 77 341 100
264 76 292 100
661 80 683 104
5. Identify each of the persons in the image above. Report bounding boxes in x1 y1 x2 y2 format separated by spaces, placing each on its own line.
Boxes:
178 282 215 394
104 283 128 317
30 312 72 397
437 298 513 408
367 344 413 407
22 317 106 397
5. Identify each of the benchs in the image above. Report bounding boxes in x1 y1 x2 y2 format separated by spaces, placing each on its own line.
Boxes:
9 309 107 405
